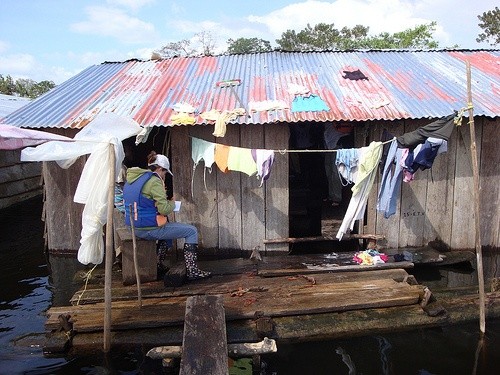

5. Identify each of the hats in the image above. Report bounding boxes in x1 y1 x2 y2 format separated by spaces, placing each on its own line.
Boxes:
148 154 173 176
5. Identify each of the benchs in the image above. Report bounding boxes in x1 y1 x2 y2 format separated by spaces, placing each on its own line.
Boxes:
114 227 161 285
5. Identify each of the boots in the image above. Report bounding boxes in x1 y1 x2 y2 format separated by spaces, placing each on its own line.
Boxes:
157 240 170 274
183 242 213 280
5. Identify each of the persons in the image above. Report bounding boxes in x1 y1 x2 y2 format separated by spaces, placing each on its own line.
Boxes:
122 152 214 282
